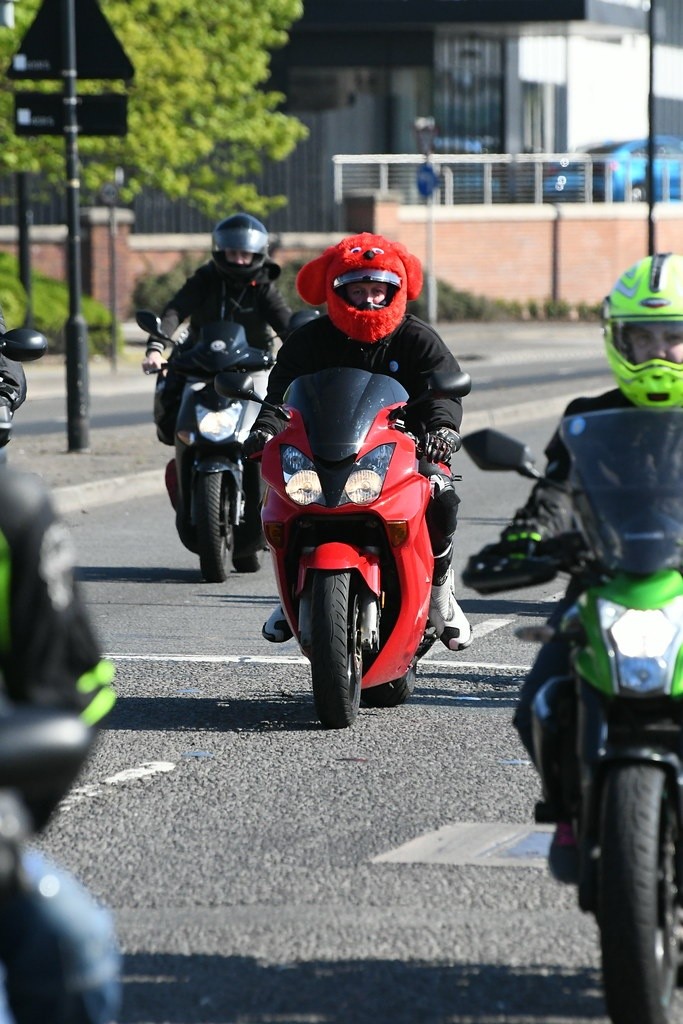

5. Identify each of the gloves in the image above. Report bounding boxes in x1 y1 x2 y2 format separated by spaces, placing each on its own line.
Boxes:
461 525 541 595
243 427 274 463
0 395 12 408
415 427 462 464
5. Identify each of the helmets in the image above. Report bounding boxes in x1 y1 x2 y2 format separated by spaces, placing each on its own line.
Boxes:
601 253 683 409
333 268 402 310
211 214 269 277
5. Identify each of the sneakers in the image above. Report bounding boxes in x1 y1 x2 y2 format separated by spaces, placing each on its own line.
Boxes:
262 604 293 643
428 569 473 651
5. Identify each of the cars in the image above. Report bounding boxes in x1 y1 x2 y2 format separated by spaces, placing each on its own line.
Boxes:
541 135 682 204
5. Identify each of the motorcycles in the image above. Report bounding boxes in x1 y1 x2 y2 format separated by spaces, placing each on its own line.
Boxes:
0 327 48 470
209 367 472 728
459 404 683 1024
133 308 317 586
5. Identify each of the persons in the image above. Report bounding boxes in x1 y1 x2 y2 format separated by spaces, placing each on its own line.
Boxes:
463 251 683 885
242 233 472 651
143 214 294 573
0 466 124 1024
0 306 26 412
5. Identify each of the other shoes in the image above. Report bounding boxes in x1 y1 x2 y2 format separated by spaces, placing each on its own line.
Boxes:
549 822 577 883
